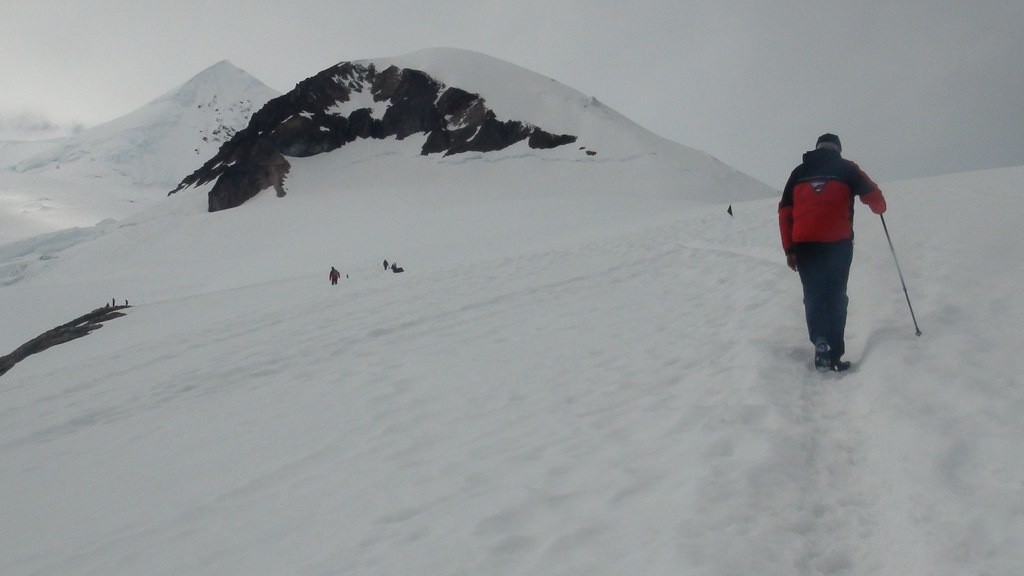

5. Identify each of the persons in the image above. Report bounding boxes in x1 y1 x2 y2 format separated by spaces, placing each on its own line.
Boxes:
125 300 128 305
329 267 340 285
113 298 115 307
383 260 388 270
779 133 887 373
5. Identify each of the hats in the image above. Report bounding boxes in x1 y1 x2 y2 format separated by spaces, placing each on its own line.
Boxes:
816 133 842 151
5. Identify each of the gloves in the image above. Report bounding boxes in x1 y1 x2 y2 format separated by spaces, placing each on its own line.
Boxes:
786 255 795 271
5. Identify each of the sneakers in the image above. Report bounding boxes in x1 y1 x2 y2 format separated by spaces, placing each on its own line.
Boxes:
830 361 851 371
815 336 831 373
